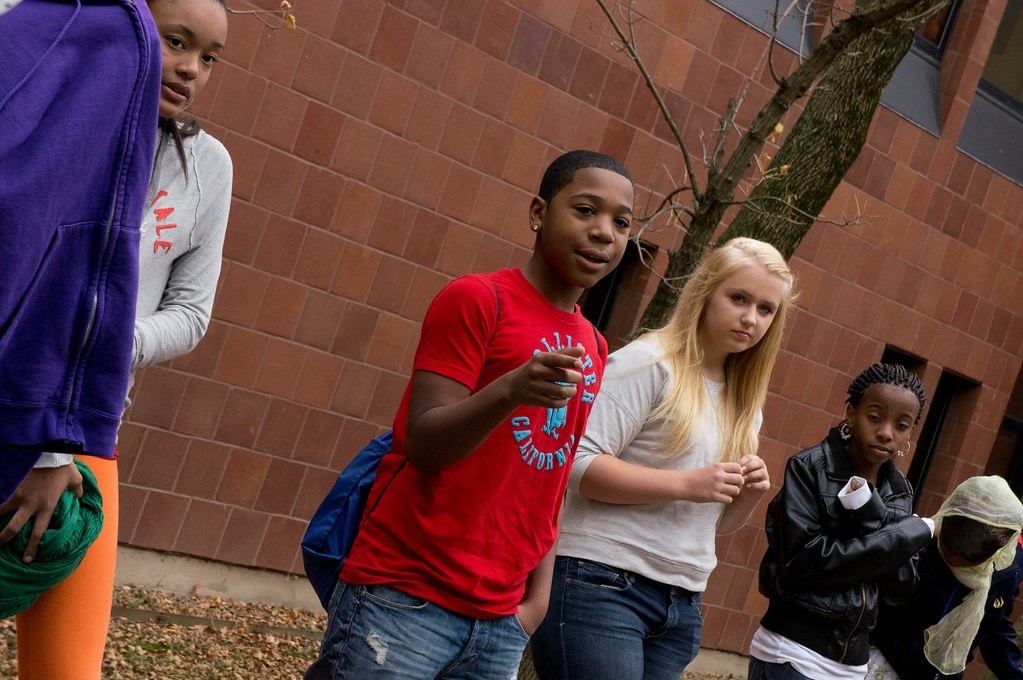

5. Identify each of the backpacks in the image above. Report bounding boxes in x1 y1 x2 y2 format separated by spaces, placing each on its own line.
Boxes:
301 273 600 609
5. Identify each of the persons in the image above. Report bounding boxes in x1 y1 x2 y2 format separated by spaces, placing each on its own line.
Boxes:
0 1 234 676
748 363 934 680
874 475 1023 680
302 148 636 679
528 237 799 680
